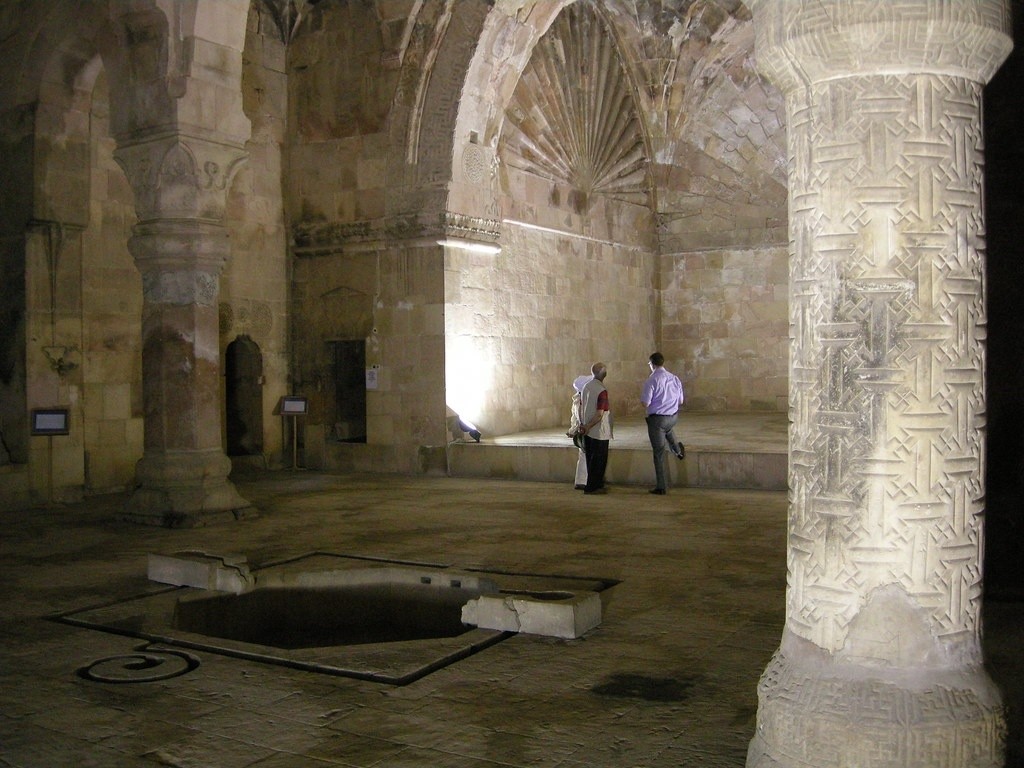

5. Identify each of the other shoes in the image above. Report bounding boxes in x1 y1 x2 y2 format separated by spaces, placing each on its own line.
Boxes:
574 484 588 490
584 487 608 494
677 442 684 460
652 488 666 495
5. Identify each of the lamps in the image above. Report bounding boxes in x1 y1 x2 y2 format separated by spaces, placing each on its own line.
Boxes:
458 416 481 443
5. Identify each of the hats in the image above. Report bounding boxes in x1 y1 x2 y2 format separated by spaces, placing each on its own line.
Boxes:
572 375 592 392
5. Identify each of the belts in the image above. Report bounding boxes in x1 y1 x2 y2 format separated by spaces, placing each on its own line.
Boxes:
649 410 678 417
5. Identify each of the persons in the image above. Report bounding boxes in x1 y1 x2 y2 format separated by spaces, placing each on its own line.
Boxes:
641 352 686 495
565 362 613 494
223 331 267 447
